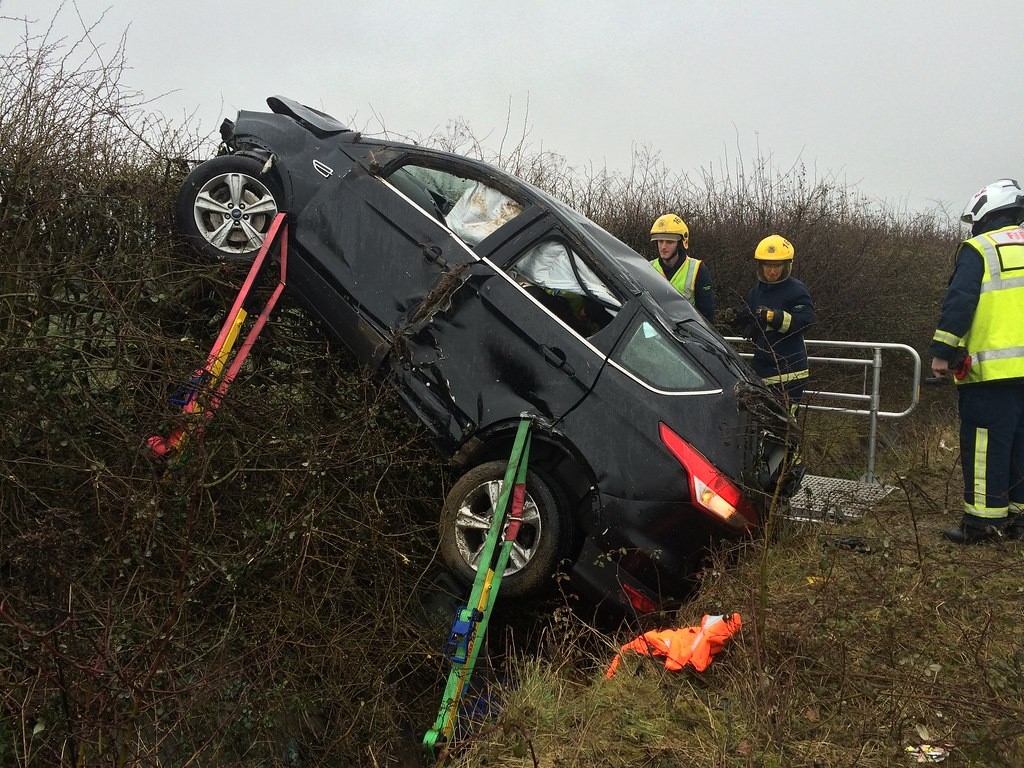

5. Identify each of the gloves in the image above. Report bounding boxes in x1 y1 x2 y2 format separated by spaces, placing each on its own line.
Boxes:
751 304 774 324
947 346 972 381
725 308 741 324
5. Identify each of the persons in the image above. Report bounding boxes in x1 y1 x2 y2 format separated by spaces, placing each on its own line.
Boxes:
932 180 1024 544
645 212 715 329
731 235 811 485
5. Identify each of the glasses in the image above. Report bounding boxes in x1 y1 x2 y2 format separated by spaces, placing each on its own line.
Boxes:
760 262 784 270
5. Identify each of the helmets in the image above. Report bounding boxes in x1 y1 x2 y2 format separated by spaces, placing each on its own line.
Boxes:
960 178 1024 222
754 235 794 284
650 214 689 249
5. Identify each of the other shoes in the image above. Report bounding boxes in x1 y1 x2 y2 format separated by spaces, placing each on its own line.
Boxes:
1003 512 1024 539
943 522 1004 544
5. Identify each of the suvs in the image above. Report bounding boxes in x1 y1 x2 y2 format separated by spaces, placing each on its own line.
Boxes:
173 96 806 629
